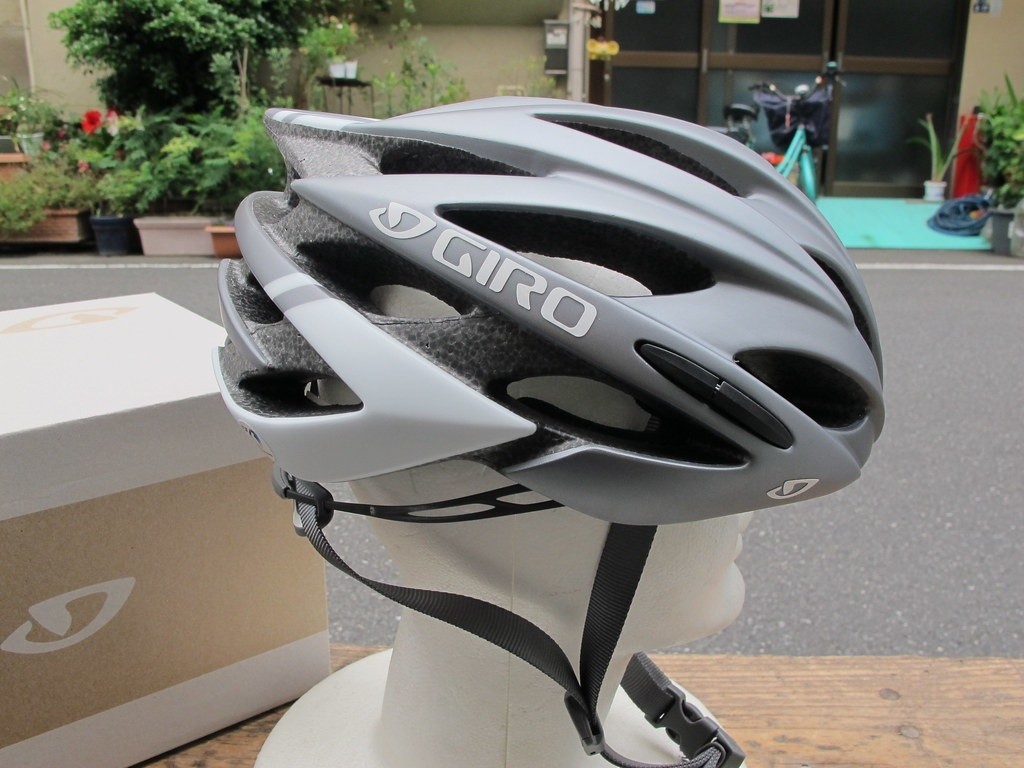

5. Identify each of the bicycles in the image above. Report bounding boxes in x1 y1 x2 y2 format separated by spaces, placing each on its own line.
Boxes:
709 61 848 208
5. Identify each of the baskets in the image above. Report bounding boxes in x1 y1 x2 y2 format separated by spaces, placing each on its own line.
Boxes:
763 99 834 149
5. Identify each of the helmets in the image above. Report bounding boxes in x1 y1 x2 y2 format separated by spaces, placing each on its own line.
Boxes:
214 96 884 525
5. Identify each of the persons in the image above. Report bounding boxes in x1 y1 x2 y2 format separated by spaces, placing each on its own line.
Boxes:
201 88 893 768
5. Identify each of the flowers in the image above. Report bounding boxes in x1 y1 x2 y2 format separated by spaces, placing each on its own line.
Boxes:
42 124 90 173
81 111 123 158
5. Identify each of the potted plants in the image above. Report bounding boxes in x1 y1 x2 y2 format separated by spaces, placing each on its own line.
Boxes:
904 113 970 201
974 102 1024 256
0 75 285 258
299 13 361 79
